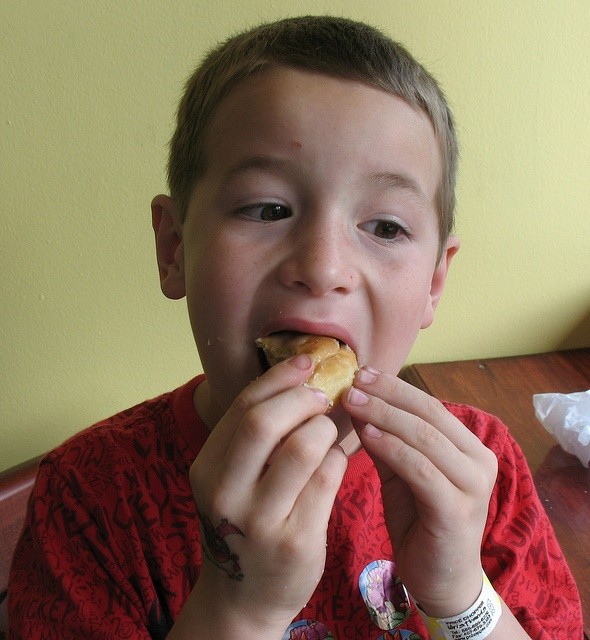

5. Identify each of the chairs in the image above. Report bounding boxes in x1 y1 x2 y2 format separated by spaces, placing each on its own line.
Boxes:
0 440 53 638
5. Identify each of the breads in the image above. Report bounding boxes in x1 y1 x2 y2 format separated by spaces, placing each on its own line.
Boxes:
255 332 359 418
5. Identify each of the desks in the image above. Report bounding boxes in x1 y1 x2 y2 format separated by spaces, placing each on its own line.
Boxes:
405 345 589 636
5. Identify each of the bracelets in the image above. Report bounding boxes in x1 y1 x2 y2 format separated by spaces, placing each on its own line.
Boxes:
413 563 503 640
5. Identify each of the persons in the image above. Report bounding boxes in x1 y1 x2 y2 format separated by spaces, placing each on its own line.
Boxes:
7 16 584 640
